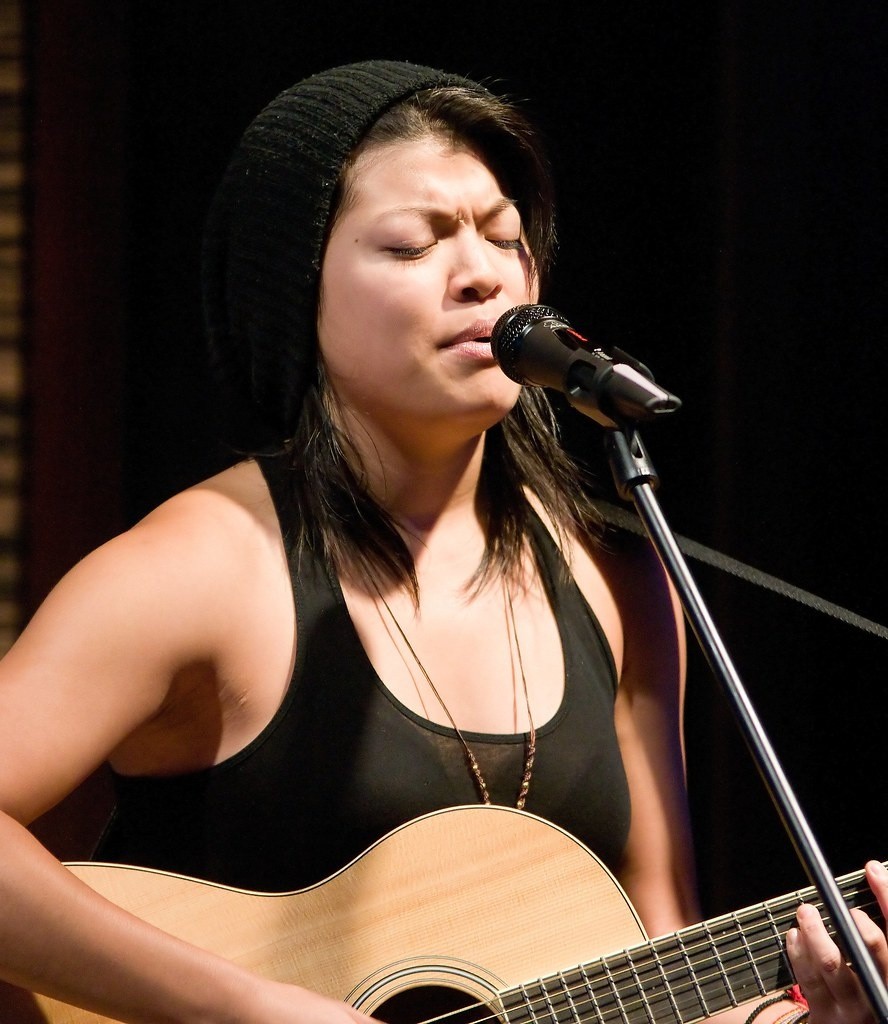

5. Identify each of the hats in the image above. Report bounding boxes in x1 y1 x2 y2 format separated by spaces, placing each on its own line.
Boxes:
198 65 496 399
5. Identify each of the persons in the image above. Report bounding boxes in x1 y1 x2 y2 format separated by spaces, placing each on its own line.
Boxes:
0 59 888 1024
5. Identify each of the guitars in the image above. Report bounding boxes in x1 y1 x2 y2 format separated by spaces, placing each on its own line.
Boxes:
0 798 888 1023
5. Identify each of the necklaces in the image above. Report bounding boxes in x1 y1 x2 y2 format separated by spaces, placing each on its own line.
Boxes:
358 554 536 810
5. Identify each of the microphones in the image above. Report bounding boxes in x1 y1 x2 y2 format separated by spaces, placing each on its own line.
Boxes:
490 303 681 415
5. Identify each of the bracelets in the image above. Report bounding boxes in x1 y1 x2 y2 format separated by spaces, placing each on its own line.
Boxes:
743 983 810 1024
